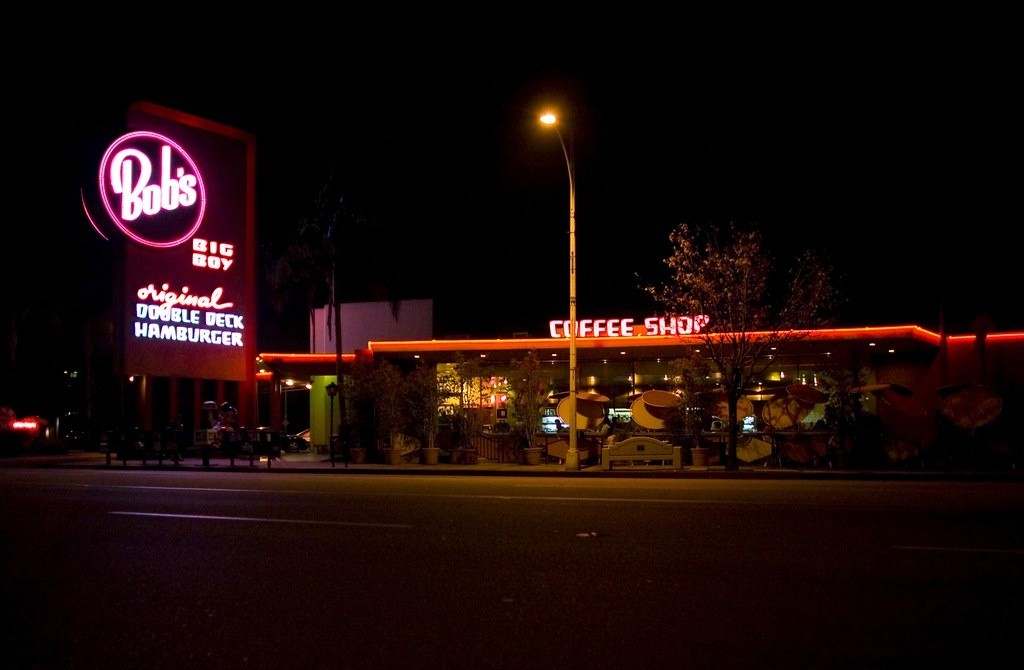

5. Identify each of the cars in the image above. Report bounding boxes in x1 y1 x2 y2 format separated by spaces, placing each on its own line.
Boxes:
284 427 311 453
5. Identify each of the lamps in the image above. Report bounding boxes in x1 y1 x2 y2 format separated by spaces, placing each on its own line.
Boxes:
326 383 339 396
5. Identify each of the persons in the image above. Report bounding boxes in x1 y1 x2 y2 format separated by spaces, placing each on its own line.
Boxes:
611 417 624 429
555 420 565 432
714 422 722 432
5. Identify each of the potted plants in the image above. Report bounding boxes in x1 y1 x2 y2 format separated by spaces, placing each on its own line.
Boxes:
339 352 496 467
668 347 711 467
509 353 550 466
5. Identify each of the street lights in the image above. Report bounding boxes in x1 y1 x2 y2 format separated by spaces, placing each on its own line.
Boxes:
536 104 581 473
